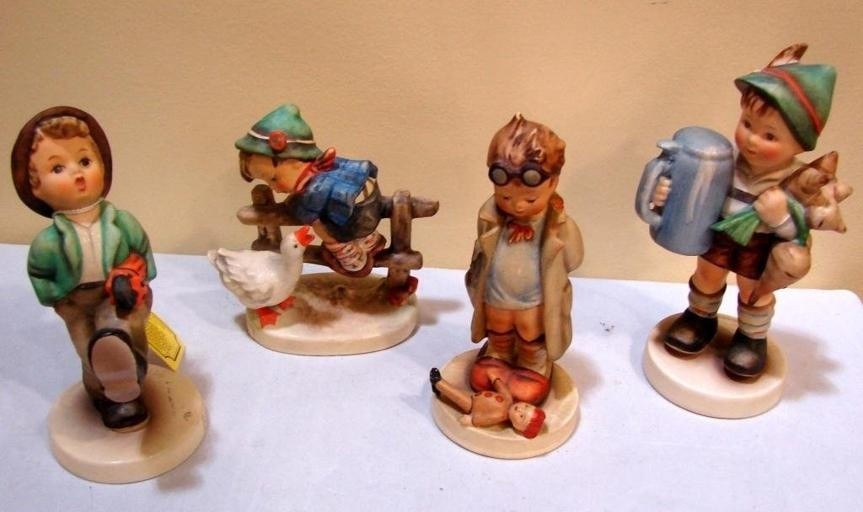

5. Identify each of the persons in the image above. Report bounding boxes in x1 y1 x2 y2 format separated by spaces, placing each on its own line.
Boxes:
465 114 584 406
10 106 158 434
430 368 545 439
652 42 836 381
234 104 386 278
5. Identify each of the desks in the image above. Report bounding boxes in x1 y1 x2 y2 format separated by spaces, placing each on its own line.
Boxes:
0 243 862 511
